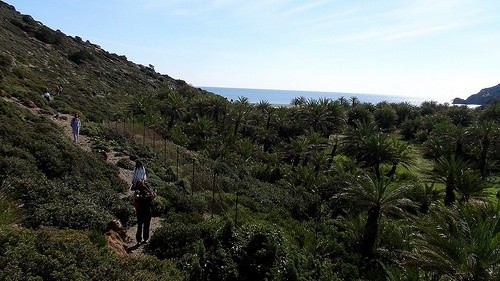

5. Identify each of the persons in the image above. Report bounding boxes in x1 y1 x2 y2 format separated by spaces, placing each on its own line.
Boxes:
55 84 63 95
133 160 146 187
71 114 81 142
134 180 155 244
44 91 50 102
